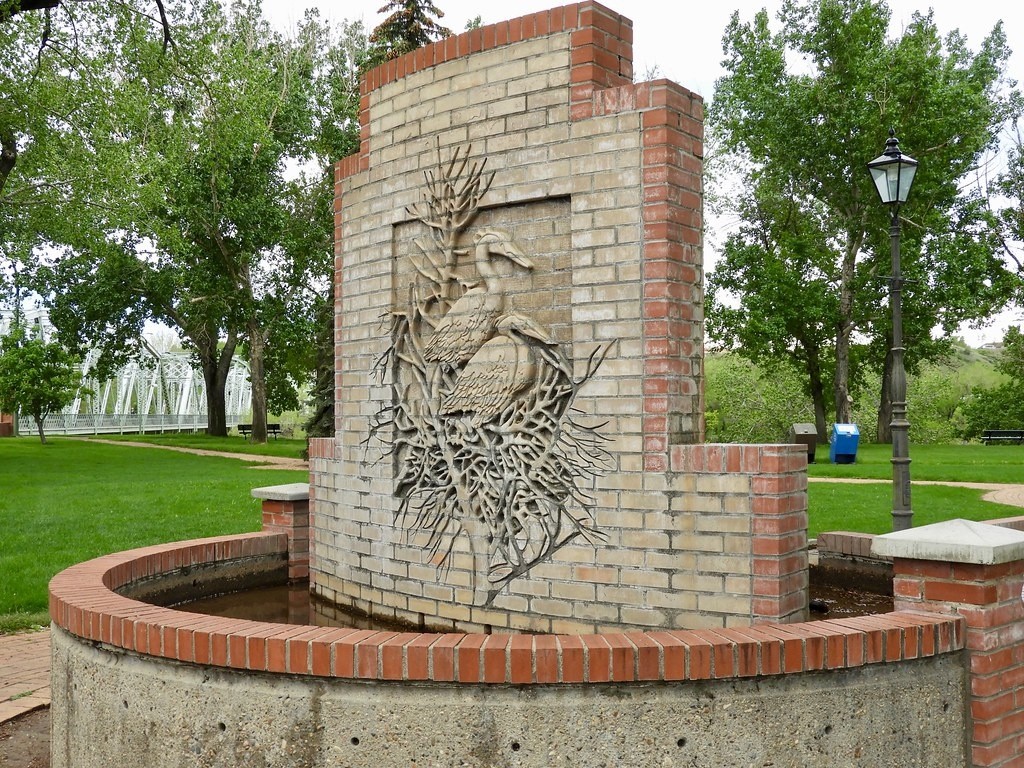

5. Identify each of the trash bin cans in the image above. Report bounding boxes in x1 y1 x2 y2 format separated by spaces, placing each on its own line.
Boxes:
790 422 819 464
829 423 861 464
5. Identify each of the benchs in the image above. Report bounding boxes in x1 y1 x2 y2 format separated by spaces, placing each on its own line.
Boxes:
980 430 1024 446
237 424 281 440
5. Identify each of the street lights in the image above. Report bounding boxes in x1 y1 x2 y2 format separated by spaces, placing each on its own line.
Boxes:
866 130 923 536
